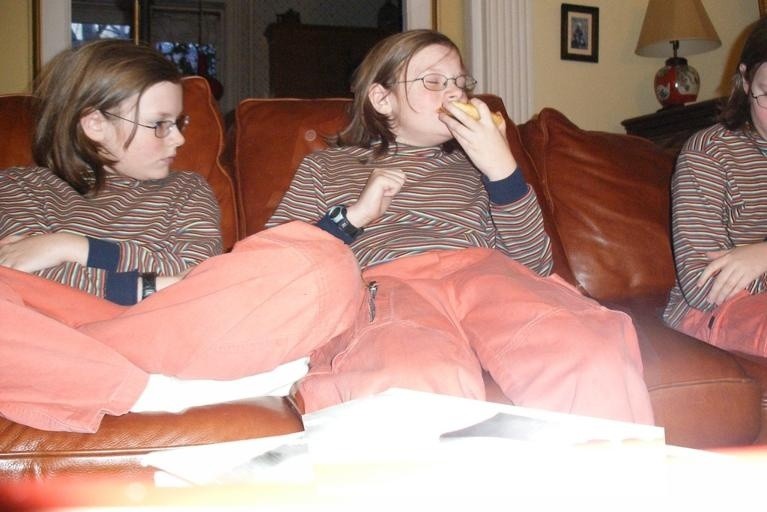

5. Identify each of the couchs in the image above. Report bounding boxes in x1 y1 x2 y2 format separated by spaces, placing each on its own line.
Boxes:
0 76 763 510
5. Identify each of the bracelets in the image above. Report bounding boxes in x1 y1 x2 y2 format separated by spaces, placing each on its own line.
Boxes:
141 270 157 303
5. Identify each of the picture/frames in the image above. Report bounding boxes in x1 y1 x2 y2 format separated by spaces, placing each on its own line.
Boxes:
559 3 598 63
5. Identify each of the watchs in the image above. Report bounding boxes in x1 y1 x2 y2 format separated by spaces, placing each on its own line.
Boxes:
326 201 364 239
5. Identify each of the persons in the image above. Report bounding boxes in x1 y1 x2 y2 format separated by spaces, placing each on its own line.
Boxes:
0 33 367 434
661 12 767 363
264 24 659 445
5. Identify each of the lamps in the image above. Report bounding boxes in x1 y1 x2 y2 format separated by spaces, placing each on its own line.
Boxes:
633 0 723 114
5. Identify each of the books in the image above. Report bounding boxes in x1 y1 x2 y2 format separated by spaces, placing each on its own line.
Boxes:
297 384 666 458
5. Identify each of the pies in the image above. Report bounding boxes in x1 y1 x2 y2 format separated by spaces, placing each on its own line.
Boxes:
449 101 504 127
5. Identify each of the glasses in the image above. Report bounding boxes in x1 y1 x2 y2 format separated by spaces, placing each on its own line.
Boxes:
388 73 478 92
97 108 191 138
746 76 767 109
